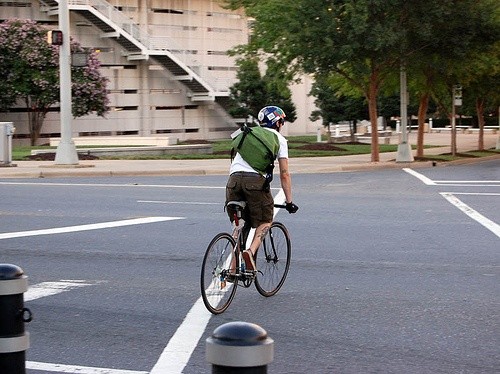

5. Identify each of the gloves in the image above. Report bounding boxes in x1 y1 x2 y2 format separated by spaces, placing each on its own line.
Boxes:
286 201 298 213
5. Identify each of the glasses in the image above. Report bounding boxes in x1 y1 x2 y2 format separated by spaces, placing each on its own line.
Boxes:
280 119 285 126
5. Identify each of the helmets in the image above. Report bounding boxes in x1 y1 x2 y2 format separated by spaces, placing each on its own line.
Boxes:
257 106 287 128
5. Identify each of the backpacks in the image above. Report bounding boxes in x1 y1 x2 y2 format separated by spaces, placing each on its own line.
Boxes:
232 124 280 179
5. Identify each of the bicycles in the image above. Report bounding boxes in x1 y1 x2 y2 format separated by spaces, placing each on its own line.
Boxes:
199 201 295 314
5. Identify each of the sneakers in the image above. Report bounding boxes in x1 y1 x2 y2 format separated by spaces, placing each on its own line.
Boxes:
244 249 257 272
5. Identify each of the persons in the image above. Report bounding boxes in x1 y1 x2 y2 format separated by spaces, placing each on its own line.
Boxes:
224 106 293 282
